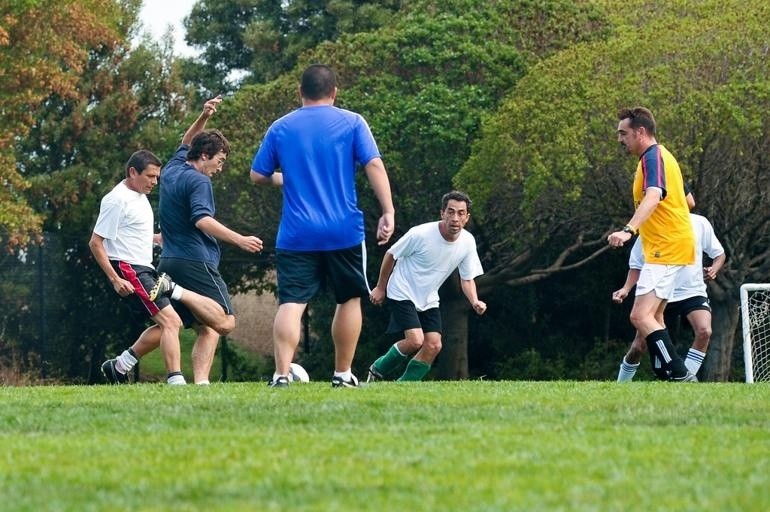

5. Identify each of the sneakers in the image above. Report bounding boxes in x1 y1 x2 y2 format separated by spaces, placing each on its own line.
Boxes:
100 359 126 385
331 371 360 388
271 371 293 389
366 363 385 382
149 271 172 302
671 370 699 385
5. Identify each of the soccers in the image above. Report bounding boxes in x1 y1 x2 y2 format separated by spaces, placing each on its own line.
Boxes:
273 363 309 382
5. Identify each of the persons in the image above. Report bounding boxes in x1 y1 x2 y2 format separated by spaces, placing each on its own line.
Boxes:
149 94 263 386
250 65 395 389
89 148 188 385
607 107 726 384
367 191 487 382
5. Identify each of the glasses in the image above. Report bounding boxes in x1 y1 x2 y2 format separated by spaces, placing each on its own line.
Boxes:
216 158 225 168
